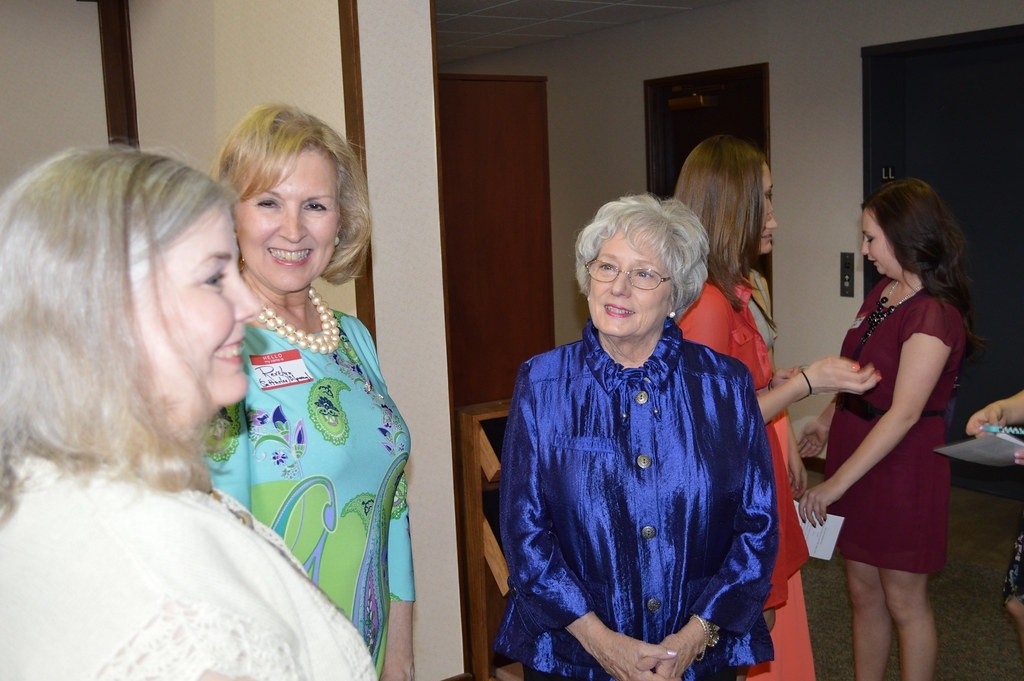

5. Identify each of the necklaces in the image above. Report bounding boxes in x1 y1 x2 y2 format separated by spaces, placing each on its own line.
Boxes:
257 286 340 354
861 280 921 344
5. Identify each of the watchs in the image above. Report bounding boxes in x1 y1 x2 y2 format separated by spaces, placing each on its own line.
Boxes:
705 619 720 647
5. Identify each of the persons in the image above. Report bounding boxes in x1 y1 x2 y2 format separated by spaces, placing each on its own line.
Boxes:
200 103 416 680
797 180 985 681
494 196 779 680
0 148 377 681
673 134 882 681
966 391 1024 652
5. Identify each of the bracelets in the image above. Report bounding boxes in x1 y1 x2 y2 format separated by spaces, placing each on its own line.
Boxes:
690 615 708 661
801 371 814 397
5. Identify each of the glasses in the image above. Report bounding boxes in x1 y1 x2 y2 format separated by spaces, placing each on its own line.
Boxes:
585 260 673 290
